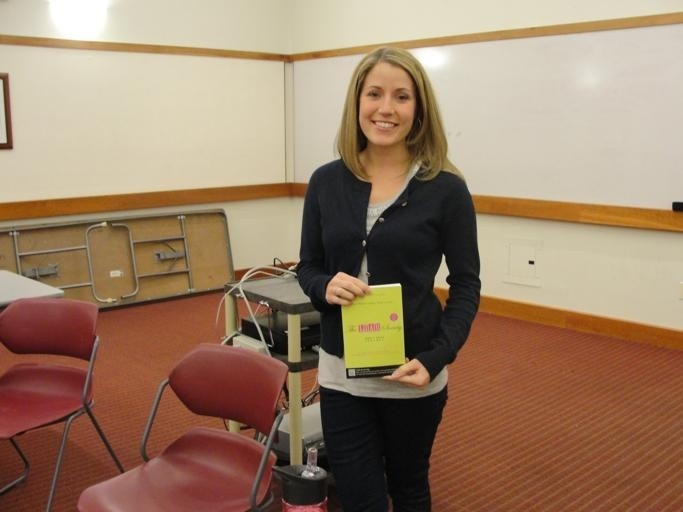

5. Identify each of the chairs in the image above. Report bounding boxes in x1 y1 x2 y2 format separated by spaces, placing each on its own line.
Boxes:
0 296 126 512
70 342 290 512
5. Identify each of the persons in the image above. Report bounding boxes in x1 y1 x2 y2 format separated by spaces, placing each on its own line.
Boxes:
294 44 481 512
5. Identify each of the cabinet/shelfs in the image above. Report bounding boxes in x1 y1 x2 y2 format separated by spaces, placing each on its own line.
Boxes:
216 272 324 467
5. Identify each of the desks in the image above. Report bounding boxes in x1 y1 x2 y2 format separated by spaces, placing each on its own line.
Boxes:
0 267 64 314
1 207 236 313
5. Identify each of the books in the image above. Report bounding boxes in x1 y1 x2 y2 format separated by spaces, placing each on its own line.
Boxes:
341 282 406 378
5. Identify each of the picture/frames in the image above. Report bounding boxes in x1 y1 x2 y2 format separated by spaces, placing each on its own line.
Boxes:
0 72 14 151
